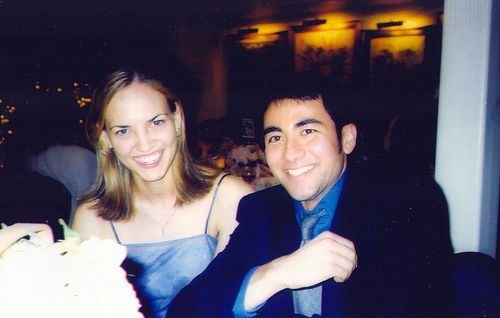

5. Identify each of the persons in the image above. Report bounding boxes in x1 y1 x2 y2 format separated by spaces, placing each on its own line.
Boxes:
165 68 459 318
69 64 254 318
0 91 438 244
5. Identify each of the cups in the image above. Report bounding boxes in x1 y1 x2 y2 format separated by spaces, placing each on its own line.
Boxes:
242 166 257 183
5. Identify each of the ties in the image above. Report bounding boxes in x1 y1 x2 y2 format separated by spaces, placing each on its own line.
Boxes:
292 210 327 317
